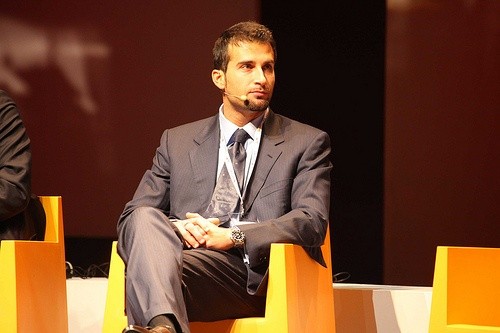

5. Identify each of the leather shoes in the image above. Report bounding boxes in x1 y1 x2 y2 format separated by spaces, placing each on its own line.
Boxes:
122 324 176 333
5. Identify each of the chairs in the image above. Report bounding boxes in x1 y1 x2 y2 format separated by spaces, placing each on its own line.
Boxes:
103 220 336 333
0 196 69 333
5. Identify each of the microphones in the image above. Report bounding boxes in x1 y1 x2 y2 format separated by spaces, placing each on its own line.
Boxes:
218 89 247 101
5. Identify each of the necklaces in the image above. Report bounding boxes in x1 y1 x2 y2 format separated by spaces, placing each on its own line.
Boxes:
220 120 258 227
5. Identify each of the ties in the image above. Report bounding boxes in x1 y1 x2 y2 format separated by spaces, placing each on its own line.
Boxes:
201 128 252 226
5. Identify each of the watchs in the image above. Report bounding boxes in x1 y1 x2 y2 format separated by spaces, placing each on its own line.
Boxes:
230 226 246 249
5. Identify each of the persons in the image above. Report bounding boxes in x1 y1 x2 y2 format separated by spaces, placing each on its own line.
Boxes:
0 88 37 245
116 22 334 333
